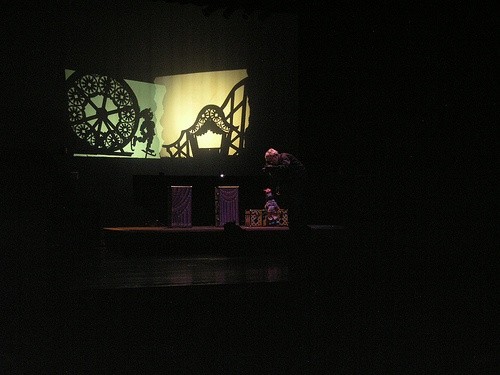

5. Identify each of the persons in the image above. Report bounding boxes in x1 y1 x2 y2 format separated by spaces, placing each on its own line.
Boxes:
264 148 312 231
